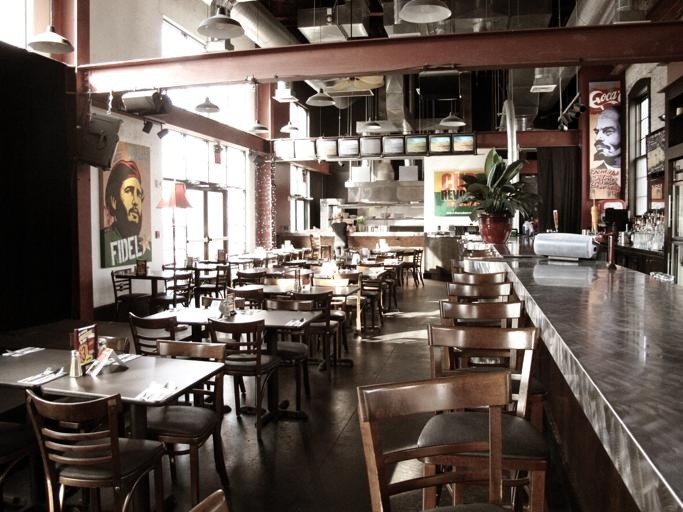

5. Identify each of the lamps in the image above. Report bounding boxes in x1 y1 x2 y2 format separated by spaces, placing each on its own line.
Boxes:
27 0 75 55
196 0 244 40
195 87 219 114
142 117 152 133
248 83 268 134
280 100 299 134
157 123 168 139
306 0 586 133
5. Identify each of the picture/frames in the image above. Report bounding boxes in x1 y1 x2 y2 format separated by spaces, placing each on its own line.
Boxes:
272 134 477 161
646 127 666 177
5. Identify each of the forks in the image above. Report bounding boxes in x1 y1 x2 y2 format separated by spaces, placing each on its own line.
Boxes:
5 346 37 355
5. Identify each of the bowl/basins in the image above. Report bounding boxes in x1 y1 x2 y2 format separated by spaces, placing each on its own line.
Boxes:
335 212 357 220
366 212 404 219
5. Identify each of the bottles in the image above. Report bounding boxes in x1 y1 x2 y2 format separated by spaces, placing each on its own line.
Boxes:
98 338 109 361
69 350 84 378
616 206 665 252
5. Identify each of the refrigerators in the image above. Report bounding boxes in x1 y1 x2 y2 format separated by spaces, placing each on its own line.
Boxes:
662 155 683 287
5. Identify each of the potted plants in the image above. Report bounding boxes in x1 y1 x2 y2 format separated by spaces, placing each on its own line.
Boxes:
449 146 544 243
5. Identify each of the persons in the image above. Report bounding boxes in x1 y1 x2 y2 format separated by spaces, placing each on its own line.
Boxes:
328 212 350 261
100 159 152 268
591 105 621 199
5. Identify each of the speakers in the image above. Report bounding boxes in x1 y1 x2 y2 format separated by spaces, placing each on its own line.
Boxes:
81 112 124 172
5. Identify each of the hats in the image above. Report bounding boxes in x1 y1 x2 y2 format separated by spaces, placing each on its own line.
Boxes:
105 160 141 216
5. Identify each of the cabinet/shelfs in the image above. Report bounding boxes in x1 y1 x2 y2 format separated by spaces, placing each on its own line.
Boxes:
656 60 683 286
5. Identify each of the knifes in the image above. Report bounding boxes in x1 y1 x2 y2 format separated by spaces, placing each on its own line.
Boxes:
27 367 60 383
